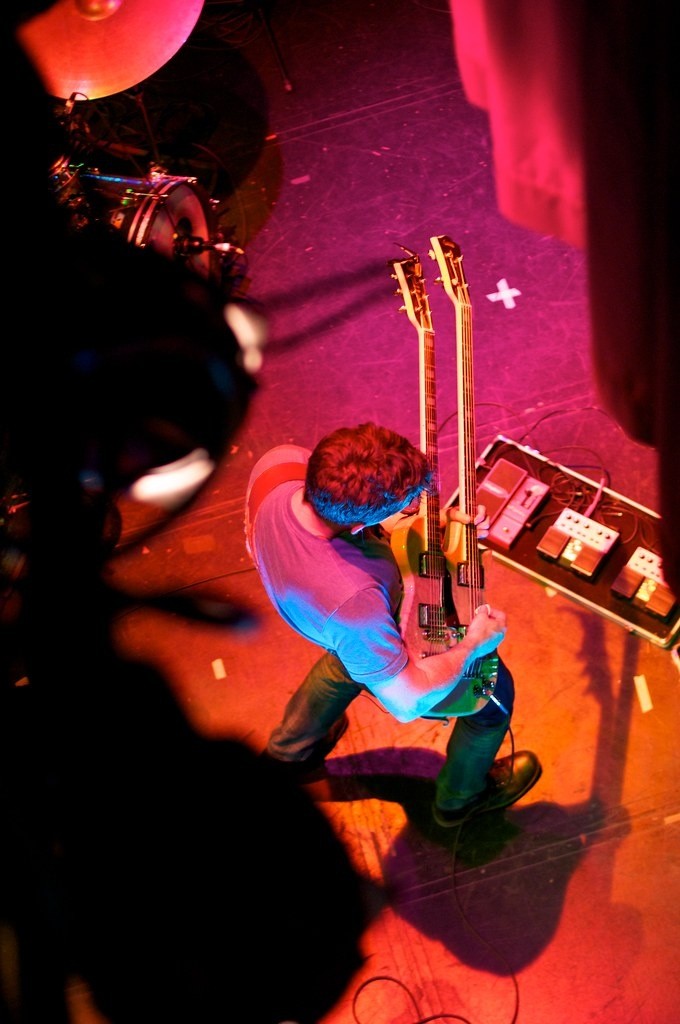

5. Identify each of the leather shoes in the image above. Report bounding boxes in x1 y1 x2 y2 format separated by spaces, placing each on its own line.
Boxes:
269 711 349 776
430 749 541 829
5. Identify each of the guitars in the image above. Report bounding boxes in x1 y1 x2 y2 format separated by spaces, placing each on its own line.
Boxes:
390 229 500 720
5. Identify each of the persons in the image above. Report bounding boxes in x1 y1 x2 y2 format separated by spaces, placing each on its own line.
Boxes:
243 422 542 828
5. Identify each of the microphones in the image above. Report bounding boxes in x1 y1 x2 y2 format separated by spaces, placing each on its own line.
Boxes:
174 236 242 255
59 92 77 129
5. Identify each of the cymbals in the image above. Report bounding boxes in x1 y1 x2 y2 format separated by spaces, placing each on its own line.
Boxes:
17 0 206 101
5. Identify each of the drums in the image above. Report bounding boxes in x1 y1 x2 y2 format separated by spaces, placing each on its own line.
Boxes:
57 167 222 282
